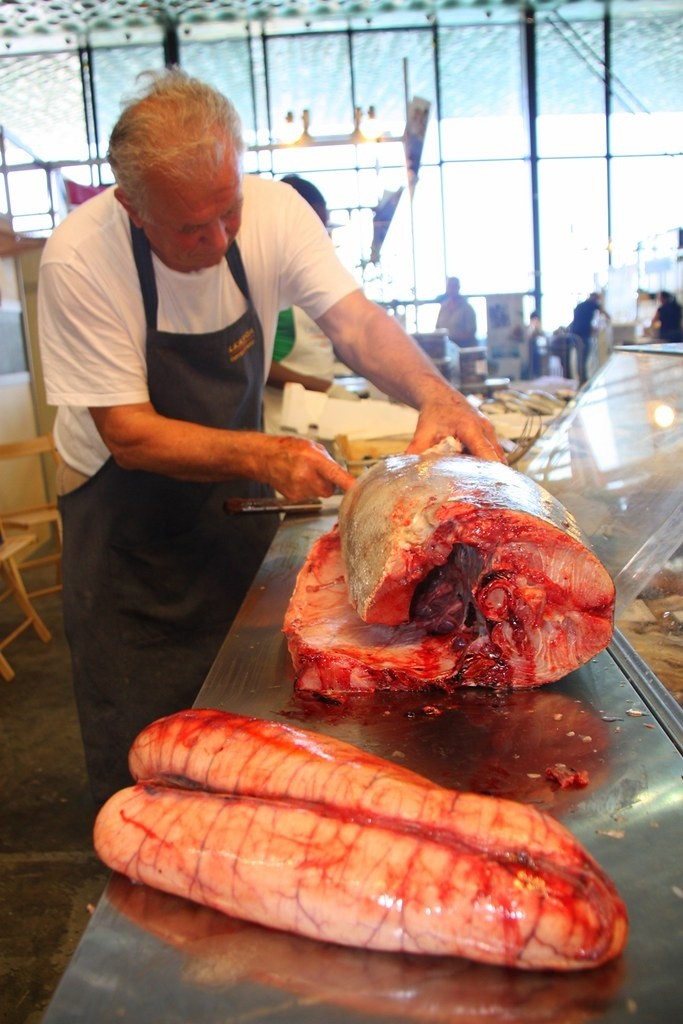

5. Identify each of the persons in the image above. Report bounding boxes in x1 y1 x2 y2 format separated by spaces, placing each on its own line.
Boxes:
649 290 683 343
34 65 506 803
262 173 397 519
434 275 479 347
519 312 548 381
569 291 612 382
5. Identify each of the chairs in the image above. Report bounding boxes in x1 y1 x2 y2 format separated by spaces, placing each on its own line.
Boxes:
0 431 60 682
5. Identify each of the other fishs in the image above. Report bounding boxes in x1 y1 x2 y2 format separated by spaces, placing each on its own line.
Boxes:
282 440 615 698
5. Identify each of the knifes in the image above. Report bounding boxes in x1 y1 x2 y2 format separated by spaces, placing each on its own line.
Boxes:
223 495 342 517
